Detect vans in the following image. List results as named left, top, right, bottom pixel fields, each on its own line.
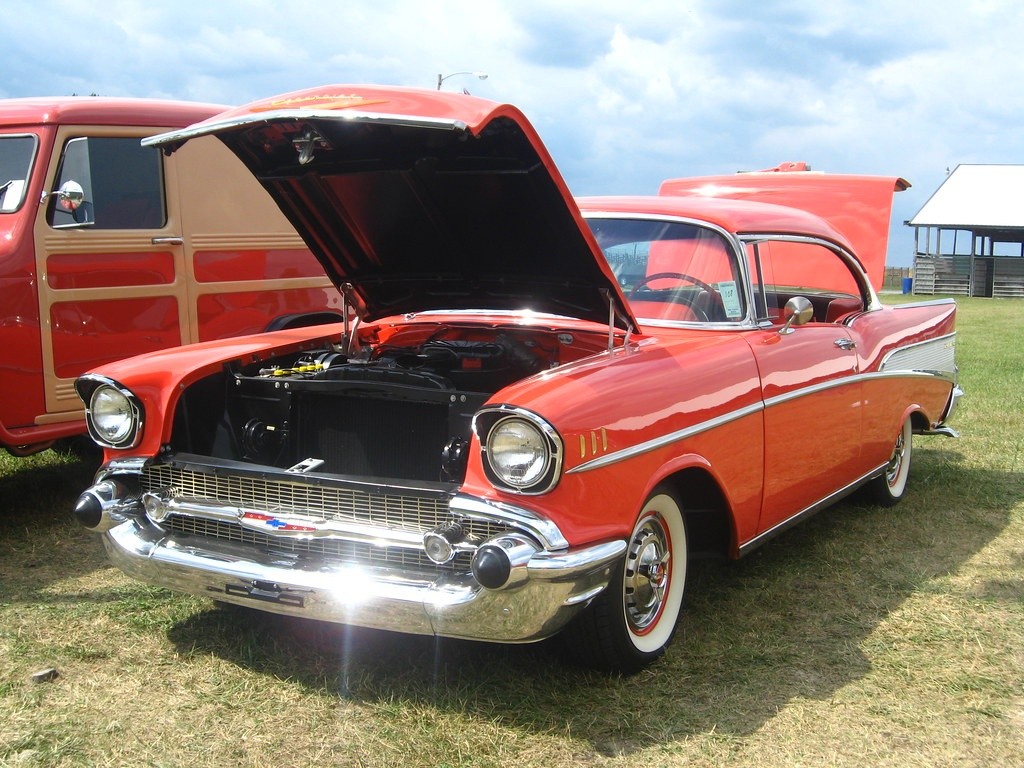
left=0, top=98, right=355, bottom=460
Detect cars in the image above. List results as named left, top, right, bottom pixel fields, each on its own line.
left=74, top=84, right=963, bottom=676
left=616, top=262, right=647, bottom=287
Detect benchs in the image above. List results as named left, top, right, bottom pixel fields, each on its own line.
left=624, top=290, right=862, bottom=322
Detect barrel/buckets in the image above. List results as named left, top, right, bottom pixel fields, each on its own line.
left=903, top=278, right=912, bottom=295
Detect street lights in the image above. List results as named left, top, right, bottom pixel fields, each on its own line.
left=436, top=71, right=489, bottom=89
